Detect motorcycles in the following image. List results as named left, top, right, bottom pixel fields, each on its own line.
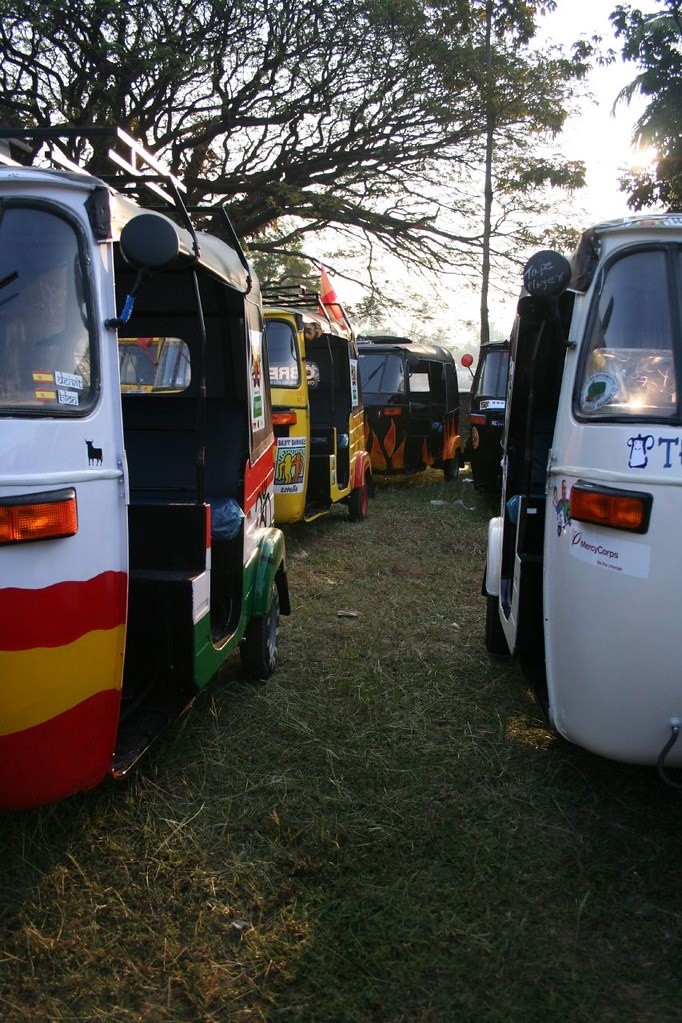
left=458, top=338, right=514, bottom=493
left=477, top=214, right=682, bottom=777
left=1, top=131, right=293, bottom=793
left=101, top=283, right=380, bottom=527
left=352, top=335, right=466, bottom=479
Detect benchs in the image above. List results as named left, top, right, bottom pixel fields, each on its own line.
left=515, top=496, right=547, bottom=654
left=128, top=503, right=210, bottom=691
left=309, top=427, right=337, bottom=504
left=121, top=394, right=247, bottom=539
left=409, top=397, right=445, bottom=463
left=508, top=391, right=560, bottom=529
left=308, top=388, right=348, bottom=450
